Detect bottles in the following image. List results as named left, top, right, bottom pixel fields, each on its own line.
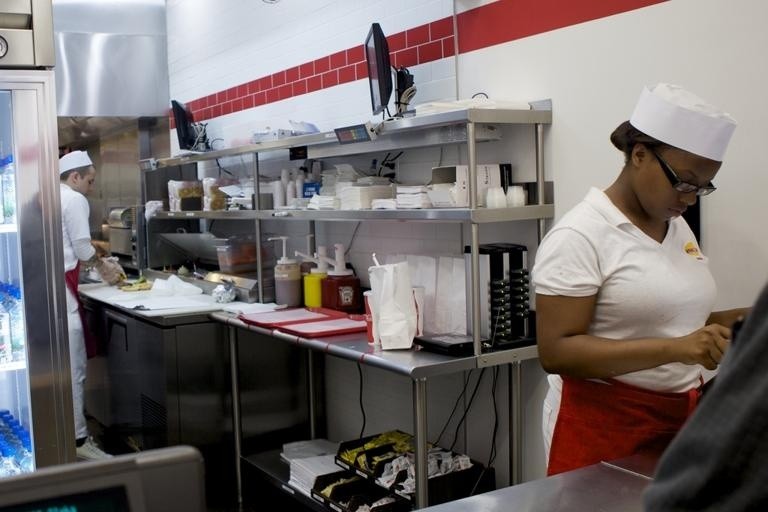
left=198, top=137, right=205, bottom=152
left=0, top=150, right=39, bottom=482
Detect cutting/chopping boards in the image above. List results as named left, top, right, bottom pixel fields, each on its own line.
left=76, top=274, right=226, bottom=317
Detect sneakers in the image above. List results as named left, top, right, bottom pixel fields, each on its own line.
left=74, top=436, right=112, bottom=463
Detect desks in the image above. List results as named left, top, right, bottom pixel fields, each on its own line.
left=409, top=462, right=654, bottom=512
left=601, top=445, right=664, bottom=480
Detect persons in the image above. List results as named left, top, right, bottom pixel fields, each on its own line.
left=529, top=81, right=737, bottom=477
left=60, top=149, right=127, bottom=463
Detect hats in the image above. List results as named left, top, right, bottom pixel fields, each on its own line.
left=630, top=84, right=740, bottom=160
left=54, top=149, right=96, bottom=174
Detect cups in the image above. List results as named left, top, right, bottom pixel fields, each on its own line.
left=270, top=163, right=322, bottom=211
left=411, top=286, right=425, bottom=338
left=362, top=287, right=383, bottom=345
left=486, top=184, right=525, bottom=209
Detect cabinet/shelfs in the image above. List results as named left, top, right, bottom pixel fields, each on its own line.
left=136, top=100, right=554, bottom=511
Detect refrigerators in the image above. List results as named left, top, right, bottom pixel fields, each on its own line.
left=0, top=65, right=84, bottom=491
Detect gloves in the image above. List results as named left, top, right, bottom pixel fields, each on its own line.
left=94, top=256, right=127, bottom=285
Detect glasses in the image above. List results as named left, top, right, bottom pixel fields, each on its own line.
left=651, top=149, right=719, bottom=196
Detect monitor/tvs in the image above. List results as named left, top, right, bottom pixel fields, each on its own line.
left=172, top=100, right=210, bottom=151
left=365, top=23, right=416, bottom=118
